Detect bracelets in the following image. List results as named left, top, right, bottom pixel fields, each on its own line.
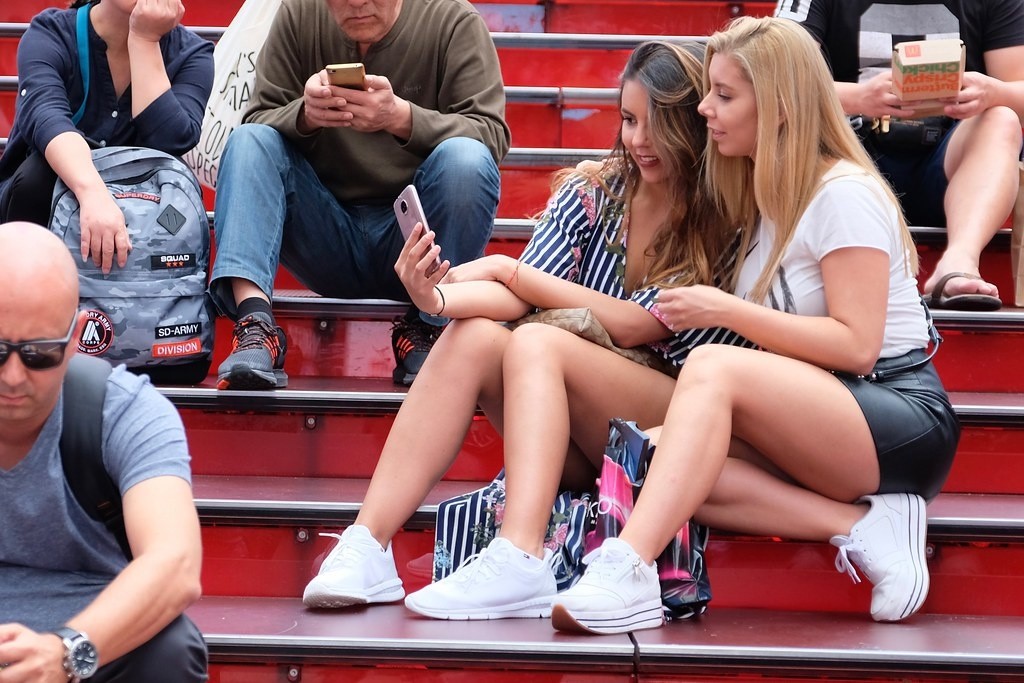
left=506, top=262, right=524, bottom=288
left=424, top=285, right=446, bottom=316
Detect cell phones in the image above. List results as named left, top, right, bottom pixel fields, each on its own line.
left=325, top=63, right=368, bottom=92
left=392, top=184, right=441, bottom=279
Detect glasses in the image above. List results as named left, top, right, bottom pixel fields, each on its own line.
left=0, top=309, right=78, bottom=370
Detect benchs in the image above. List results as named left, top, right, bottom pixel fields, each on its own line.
left=0, top=0, right=1024, bottom=683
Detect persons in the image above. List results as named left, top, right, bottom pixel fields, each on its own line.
left=551, top=15, right=963, bottom=636
left=209, top=0, right=512, bottom=391
left=0, top=0, right=215, bottom=275
left=302, top=40, right=768, bottom=621
left=0, top=222, right=209, bottom=683
left=773, top=0, right=1024, bottom=310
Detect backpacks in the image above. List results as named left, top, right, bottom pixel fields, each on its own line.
left=49, top=145, right=214, bottom=383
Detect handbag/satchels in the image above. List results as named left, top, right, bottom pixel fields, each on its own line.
left=182, top=0, right=283, bottom=190
left=432, top=466, right=590, bottom=597
left=582, top=416, right=712, bottom=622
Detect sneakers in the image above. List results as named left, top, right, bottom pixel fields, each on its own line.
left=303, top=524, right=405, bottom=607
left=216, top=315, right=289, bottom=386
left=404, top=538, right=557, bottom=619
left=831, top=492, right=930, bottom=621
left=389, top=314, right=443, bottom=383
left=551, top=538, right=664, bottom=633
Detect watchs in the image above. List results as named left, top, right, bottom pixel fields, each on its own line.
left=53, top=627, right=98, bottom=683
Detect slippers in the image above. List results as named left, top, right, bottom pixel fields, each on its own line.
left=922, top=272, right=1002, bottom=310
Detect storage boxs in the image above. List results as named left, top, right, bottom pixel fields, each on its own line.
left=892, top=38, right=967, bottom=118
